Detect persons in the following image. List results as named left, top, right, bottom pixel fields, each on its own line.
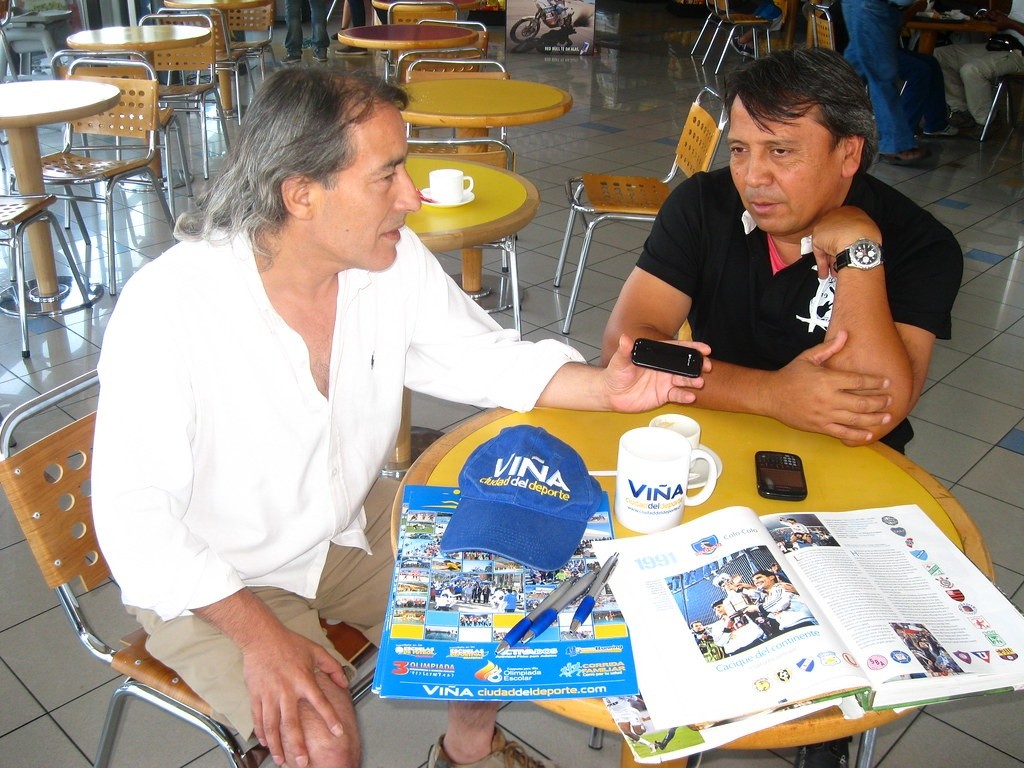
left=776, top=517, right=841, bottom=555
left=898, top=0, right=959, bottom=135
left=933, top=0, right=1024, bottom=141
left=331, top=0, right=351, bottom=40
left=601, top=45, right=964, bottom=768
left=730, top=0, right=783, bottom=58
left=690, top=563, right=817, bottom=659
left=603, top=693, right=707, bottom=754
left=335, top=0, right=388, bottom=54
left=393, top=513, right=517, bottom=626
left=280, top=0, right=330, bottom=64
left=90, top=56, right=712, bottom=767
left=524, top=514, right=592, bottom=638
left=840, top=0, right=932, bottom=165
left=894, top=624, right=962, bottom=676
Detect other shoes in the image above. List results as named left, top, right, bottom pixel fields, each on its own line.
left=331, top=33, right=339, bottom=39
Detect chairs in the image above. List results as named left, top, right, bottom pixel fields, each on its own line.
left=689, top=0, right=1024, bottom=142
left=0, top=0, right=522, bottom=359
left=553, top=87, right=730, bottom=335
left=0, top=370, right=380, bottom=768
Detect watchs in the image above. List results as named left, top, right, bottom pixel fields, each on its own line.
left=833, top=236, right=884, bottom=274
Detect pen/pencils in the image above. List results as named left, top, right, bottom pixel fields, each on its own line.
left=521, top=569, right=598, bottom=644
left=569, top=552, right=619, bottom=634
left=495, top=577, right=576, bottom=655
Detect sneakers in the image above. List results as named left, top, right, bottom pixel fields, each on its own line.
left=967, top=116, right=1002, bottom=141
left=948, top=110, right=975, bottom=128
left=923, top=124, right=959, bottom=138
left=729, top=36, right=744, bottom=54
left=741, top=45, right=755, bottom=58
left=430, top=725, right=555, bottom=768
left=313, top=50, right=327, bottom=61
left=796, top=734, right=853, bottom=768
left=879, top=147, right=931, bottom=165
left=280, top=54, right=301, bottom=64
left=335, top=45, right=368, bottom=55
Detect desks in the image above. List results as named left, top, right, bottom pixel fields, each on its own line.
left=905, top=15, right=998, bottom=55
left=1, top=0, right=571, bottom=314
left=389, top=405, right=993, bottom=768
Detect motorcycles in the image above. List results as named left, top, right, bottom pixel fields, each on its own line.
left=509, top=0, right=578, bottom=44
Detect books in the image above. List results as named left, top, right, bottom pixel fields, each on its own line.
left=591, top=502, right=1023, bottom=730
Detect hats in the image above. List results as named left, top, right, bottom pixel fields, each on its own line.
left=439, top=424, right=603, bottom=572
left=712, top=573, right=732, bottom=588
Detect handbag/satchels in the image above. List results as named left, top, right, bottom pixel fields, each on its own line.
left=986, top=33, right=1024, bottom=56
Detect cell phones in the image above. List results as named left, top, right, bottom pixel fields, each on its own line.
left=755, top=451, right=808, bottom=501
left=631, top=338, right=703, bottom=378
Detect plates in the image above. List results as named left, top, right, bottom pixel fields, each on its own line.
left=685, top=445, right=722, bottom=490
left=418, top=187, right=476, bottom=208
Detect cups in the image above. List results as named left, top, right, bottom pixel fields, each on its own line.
left=429, top=168, right=474, bottom=205
left=648, top=414, right=701, bottom=468
left=613, top=426, right=718, bottom=535
